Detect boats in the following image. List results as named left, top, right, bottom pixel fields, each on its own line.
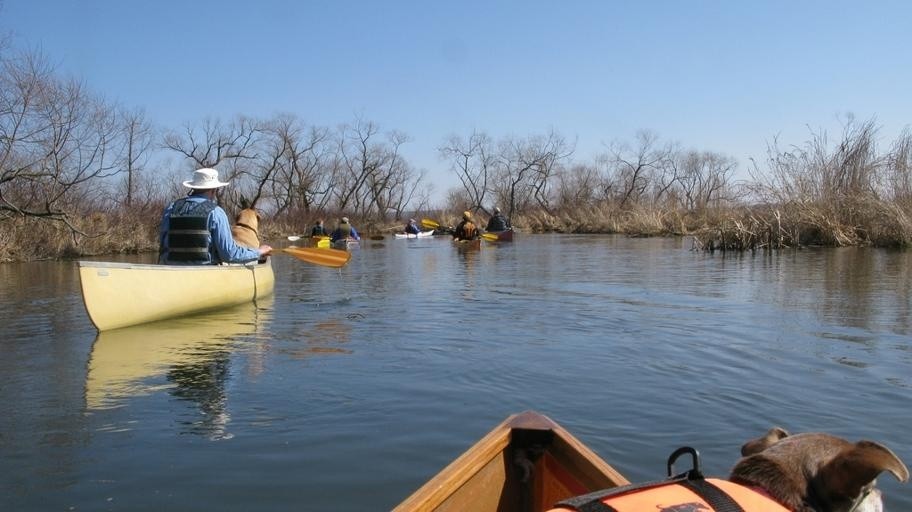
left=384, top=407, right=638, bottom=512
left=75, top=251, right=276, bottom=332
left=310, top=215, right=515, bottom=255
left=81, top=291, right=276, bottom=414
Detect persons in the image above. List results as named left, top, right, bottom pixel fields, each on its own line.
left=405, top=219, right=420, bottom=234
left=331, top=216, right=360, bottom=242
left=159, top=168, right=273, bottom=266
left=455, top=211, right=479, bottom=241
left=311, top=220, right=328, bottom=237
left=485, top=207, right=508, bottom=232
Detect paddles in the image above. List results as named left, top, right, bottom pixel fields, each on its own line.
left=318, top=237, right=359, bottom=248
left=421, top=218, right=499, bottom=242
left=268, top=248, right=353, bottom=267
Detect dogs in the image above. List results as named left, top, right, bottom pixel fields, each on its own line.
left=727, top=426, right=910, bottom=512
left=219, top=208, right=262, bottom=266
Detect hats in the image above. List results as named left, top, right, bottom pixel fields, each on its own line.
left=341, top=217, right=349, bottom=223
left=183, top=168, right=229, bottom=189
left=409, top=219, right=416, bottom=224
left=464, top=210, right=473, bottom=219
left=492, top=207, right=501, bottom=212
